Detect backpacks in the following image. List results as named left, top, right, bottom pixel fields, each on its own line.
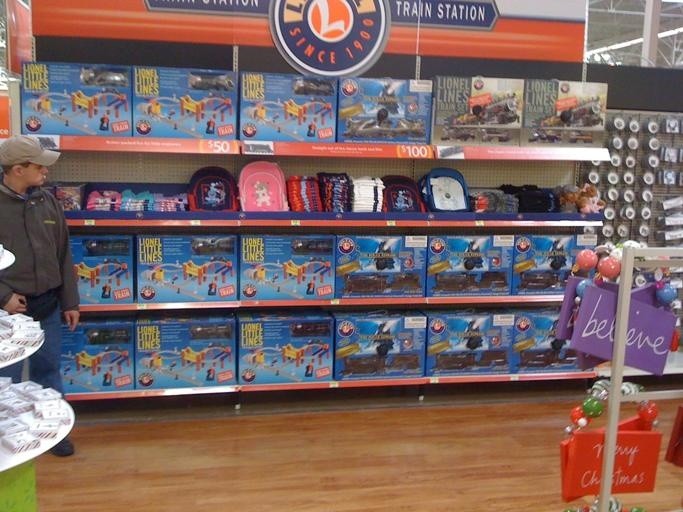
left=417, top=165, right=470, bottom=213
left=378, top=173, right=424, bottom=216
left=237, top=159, right=288, bottom=213
left=185, top=163, right=239, bottom=215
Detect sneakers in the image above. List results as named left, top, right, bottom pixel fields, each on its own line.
left=52, top=438, right=75, bottom=458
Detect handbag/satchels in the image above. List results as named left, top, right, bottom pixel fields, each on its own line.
left=21, top=289, right=64, bottom=322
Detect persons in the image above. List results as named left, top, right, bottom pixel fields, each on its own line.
left=0, top=131, right=81, bottom=457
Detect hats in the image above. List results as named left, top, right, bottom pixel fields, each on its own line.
left=0, top=135, right=59, bottom=167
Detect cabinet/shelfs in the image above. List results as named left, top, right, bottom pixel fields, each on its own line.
left=18, top=135, right=610, bottom=401
